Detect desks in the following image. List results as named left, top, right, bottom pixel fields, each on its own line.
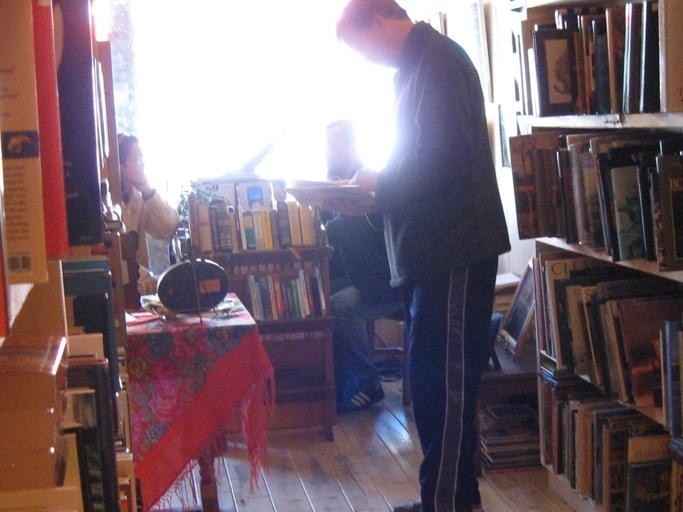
left=472, top=339, right=541, bottom=478
left=124, top=292, right=277, bottom=512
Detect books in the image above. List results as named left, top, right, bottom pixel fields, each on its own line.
left=513, top=2, right=681, bottom=117
left=509, top=131, right=682, bottom=273
left=288, top=180, right=373, bottom=205
left=479, top=245, right=681, bottom=510
left=187, top=191, right=326, bottom=321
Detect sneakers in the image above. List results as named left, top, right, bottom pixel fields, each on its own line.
left=338, top=384, right=384, bottom=413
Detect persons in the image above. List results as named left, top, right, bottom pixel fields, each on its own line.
left=337, top=0, right=512, bottom=512
left=116, top=132, right=179, bottom=295
left=318, top=120, right=405, bottom=414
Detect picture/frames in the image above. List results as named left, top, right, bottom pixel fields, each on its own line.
left=496, top=260, right=535, bottom=357
left=532, top=30, right=572, bottom=117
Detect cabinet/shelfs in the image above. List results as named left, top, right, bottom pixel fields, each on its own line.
left=0, top=0, right=140, bottom=512
left=509, top=0, right=683, bottom=512
left=171, top=238, right=337, bottom=442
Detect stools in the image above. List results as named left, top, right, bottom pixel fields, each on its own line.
left=367, top=310, right=410, bottom=405
